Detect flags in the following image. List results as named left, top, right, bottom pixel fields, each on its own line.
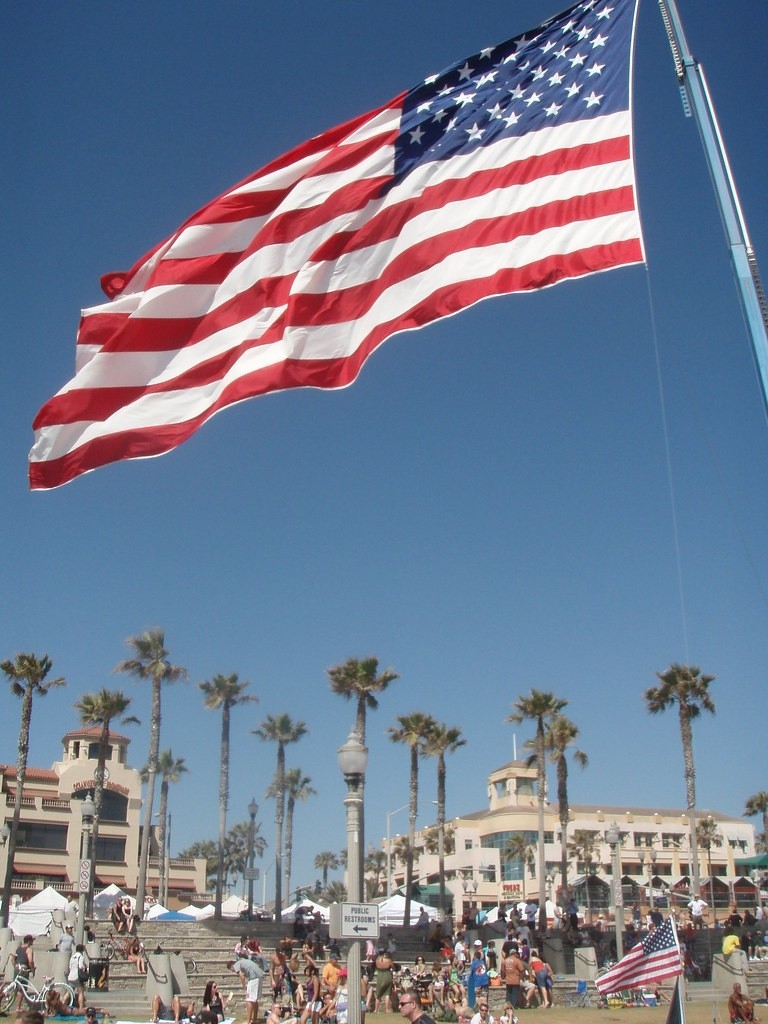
left=26, top=1, right=648, bottom=492
left=594, top=909, right=685, bottom=995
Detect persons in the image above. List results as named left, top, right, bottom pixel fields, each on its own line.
left=413, top=900, right=555, bottom=1009
left=203, top=981, right=224, bottom=1024
left=751, top=865, right=765, bottom=886
left=293, top=905, right=342, bottom=968
left=566, top=899, right=579, bottom=927
left=111, top=896, right=126, bottom=932
left=68, top=944, right=86, bottom=1010
left=41, top=990, right=116, bottom=1018
left=11, top=935, right=35, bottom=1014
left=227, top=958, right=266, bottom=1024
left=152, top=994, right=196, bottom=1024
left=499, top=1002, right=519, bottom=1024
left=470, top=1003, right=497, bottom=1024
left=266, top=936, right=342, bottom=1024
left=234, top=934, right=269, bottom=973
left=553, top=902, right=562, bottom=929
left=22, top=1011, right=44, bottom=1024
left=122, top=898, right=134, bottom=933
left=56, top=925, right=76, bottom=979
left=728, top=983, right=759, bottom=1024
left=596, top=893, right=768, bottom=1010
left=398, top=991, right=436, bottom=1024
left=64, top=894, right=79, bottom=920
left=326, top=935, right=465, bottom=1024
left=85, top=1007, right=98, bottom=1024
left=84, top=925, right=95, bottom=943
left=126, top=936, right=146, bottom=974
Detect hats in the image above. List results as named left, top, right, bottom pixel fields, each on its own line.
left=23, top=935, right=36, bottom=943
left=65, top=925, right=73, bottom=929
left=509, top=949, right=517, bottom=955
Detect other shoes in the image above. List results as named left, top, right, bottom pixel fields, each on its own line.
left=748, top=956, right=768, bottom=961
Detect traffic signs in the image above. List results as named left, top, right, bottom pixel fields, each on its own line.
left=340, top=902, right=380, bottom=938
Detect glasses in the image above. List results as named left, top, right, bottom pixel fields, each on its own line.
left=399, top=1002, right=411, bottom=1007
left=275, top=1007, right=282, bottom=1010
left=480, top=1008, right=488, bottom=1012
left=213, top=985, right=217, bottom=989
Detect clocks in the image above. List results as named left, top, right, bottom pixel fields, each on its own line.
left=94, top=766, right=110, bottom=781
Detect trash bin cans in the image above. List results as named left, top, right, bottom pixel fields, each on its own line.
left=87, top=956, right=108, bottom=992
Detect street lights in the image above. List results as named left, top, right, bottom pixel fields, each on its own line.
left=386, top=801, right=439, bottom=897
left=335, top=725, right=370, bottom=1024
left=497, top=867, right=520, bottom=909
left=606, top=825, right=624, bottom=964
left=461, top=879, right=479, bottom=909
left=262, top=854, right=285, bottom=911
left=247, top=797, right=260, bottom=922
left=75, top=790, right=97, bottom=950
left=637, top=849, right=657, bottom=908
left=154, top=809, right=171, bottom=910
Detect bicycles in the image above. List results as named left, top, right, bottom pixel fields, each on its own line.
left=99, top=926, right=146, bottom=960
left=155, top=941, right=197, bottom=975
left=0, top=962, right=75, bottom=1016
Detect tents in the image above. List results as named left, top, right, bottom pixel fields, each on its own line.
left=481, top=900, right=585, bottom=924
left=10, top=883, right=440, bottom=938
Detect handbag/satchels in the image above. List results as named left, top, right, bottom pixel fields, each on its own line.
left=389, top=990, right=400, bottom=1011
left=133, top=915, right=140, bottom=922
left=412, top=965, right=418, bottom=982
left=77, top=968, right=89, bottom=982
